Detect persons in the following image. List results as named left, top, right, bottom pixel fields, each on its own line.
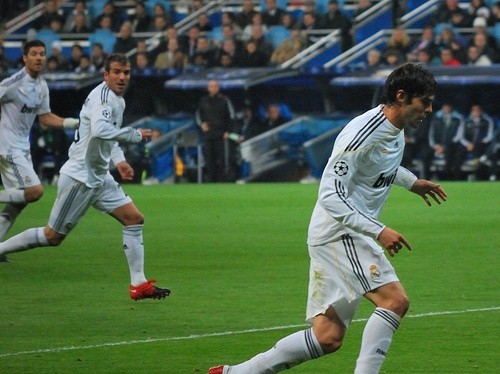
left=198, top=80, right=236, bottom=183
left=0, top=55, right=171, bottom=302
left=2, top=1, right=500, bottom=183
left=0, top=40, right=81, bottom=261
left=211, top=63, right=447, bottom=374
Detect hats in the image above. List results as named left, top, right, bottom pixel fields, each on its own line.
left=477, top=7, right=489, bottom=17
left=473, top=17, right=486, bottom=27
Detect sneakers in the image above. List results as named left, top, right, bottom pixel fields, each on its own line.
left=208, top=364, right=230, bottom=374
left=129, top=279, right=171, bottom=301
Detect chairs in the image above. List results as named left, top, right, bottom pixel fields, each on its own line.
left=0, top=0, right=500, bottom=180
